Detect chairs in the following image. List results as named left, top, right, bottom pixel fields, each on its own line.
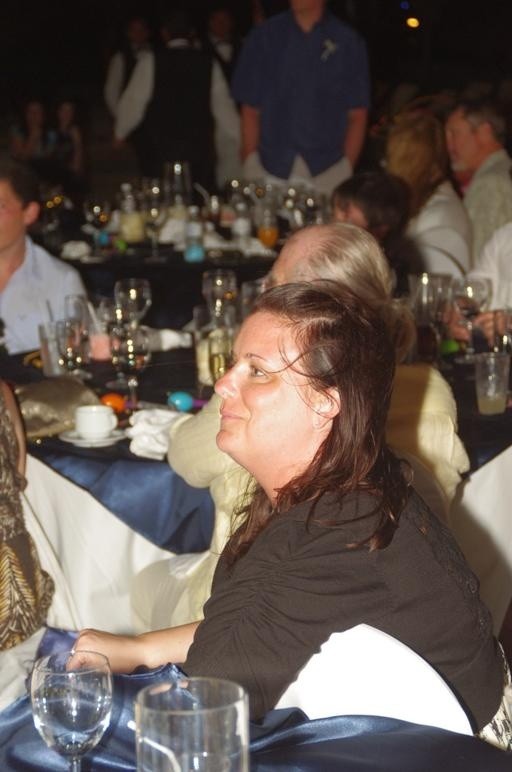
left=270, top=623, right=474, bottom=738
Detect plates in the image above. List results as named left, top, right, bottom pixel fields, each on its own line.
left=57, top=429, right=128, bottom=449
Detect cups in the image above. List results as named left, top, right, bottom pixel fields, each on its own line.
left=476, top=353, right=510, bottom=414
left=74, top=404, right=117, bottom=438
left=134, top=677, right=251, bottom=770
left=190, top=267, right=279, bottom=386
left=38, top=322, right=65, bottom=375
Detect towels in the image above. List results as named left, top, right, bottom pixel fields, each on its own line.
left=122, top=401, right=196, bottom=461
left=144, top=326, right=194, bottom=355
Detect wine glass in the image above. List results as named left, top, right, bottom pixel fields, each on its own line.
left=30, top=652, right=116, bottom=771
left=84, top=160, right=327, bottom=264
left=413, top=271, right=489, bottom=366
left=59, top=291, right=96, bottom=381
left=110, top=279, right=152, bottom=406
